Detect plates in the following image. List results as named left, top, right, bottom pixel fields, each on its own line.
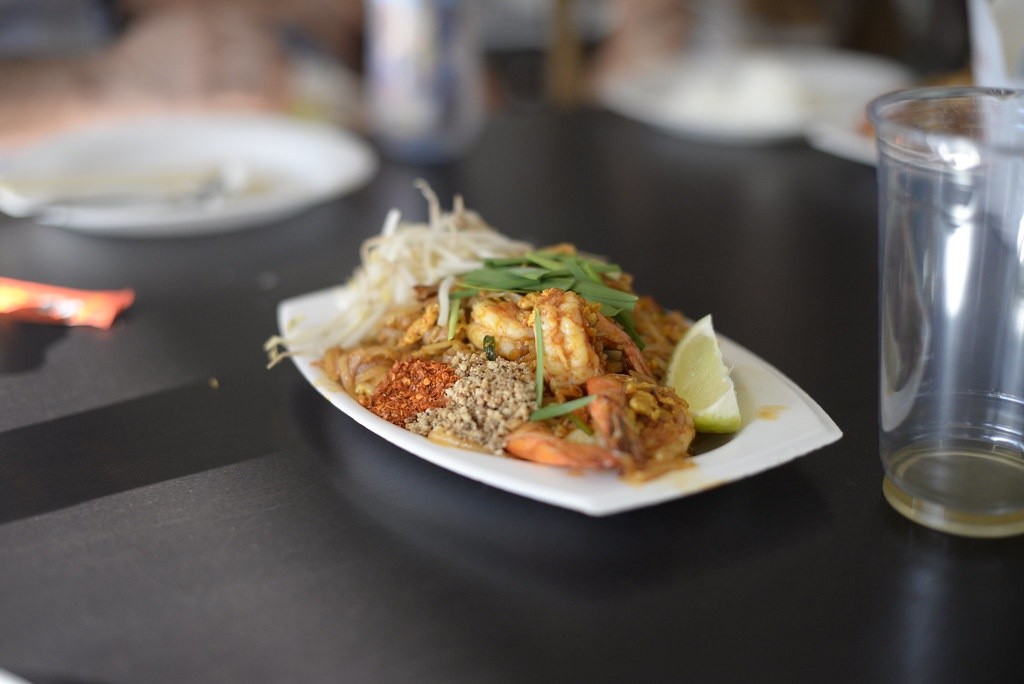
left=0, top=110, right=383, bottom=240
left=273, top=277, right=843, bottom=518
left=600, top=42, right=913, bottom=146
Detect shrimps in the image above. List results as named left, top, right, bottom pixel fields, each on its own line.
left=466, top=287, right=696, bottom=470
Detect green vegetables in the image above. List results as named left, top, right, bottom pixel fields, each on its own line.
left=435, top=244, right=647, bottom=418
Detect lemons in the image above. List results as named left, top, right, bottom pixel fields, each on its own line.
left=659, top=312, right=741, bottom=432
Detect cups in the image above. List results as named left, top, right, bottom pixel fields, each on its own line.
left=866, top=86, right=1024, bottom=537
